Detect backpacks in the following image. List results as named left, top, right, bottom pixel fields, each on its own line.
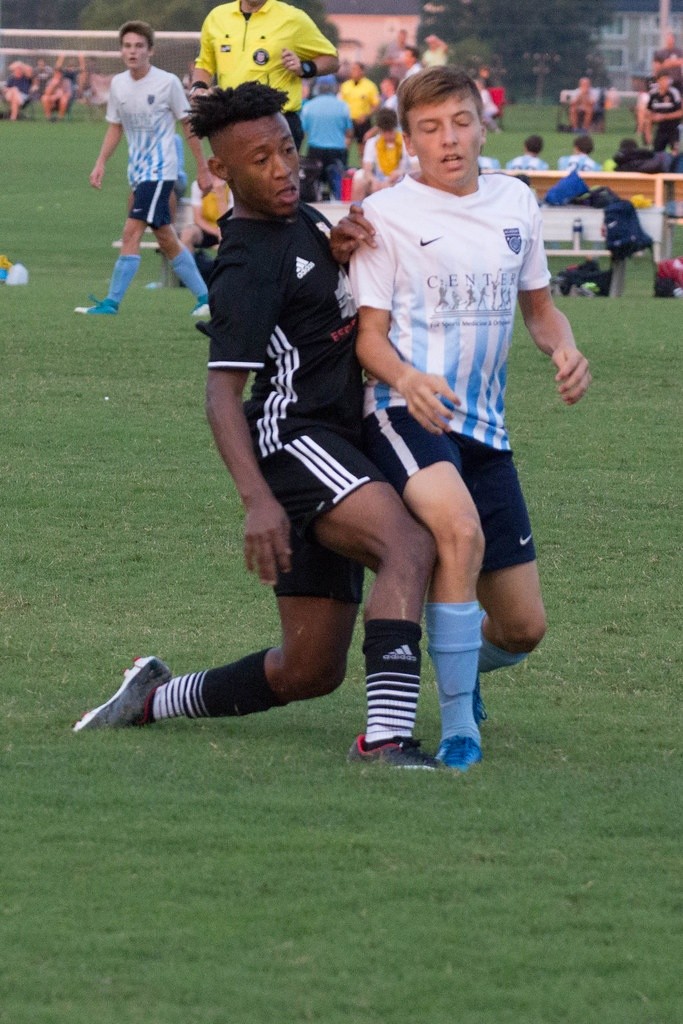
left=326, top=159, right=350, bottom=199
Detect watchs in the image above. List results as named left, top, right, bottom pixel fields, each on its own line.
left=300, top=61, right=311, bottom=78
left=189, top=86, right=198, bottom=97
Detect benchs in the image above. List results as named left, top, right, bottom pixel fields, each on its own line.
left=540, top=205, right=631, bottom=298
left=112, top=199, right=348, bottom=292
left=483, top=168, right=683, bottom=264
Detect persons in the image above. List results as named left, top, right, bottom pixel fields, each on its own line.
left=0, top=0, right=683, bottom=316
left=349, top=65, right=593, bottom=770
left=73, top=83, right=446, bottom=768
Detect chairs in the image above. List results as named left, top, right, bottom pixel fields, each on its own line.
left=491, top=86, right=507, bottom=133
left=0, top=68, right=79, bottom=120
left=556, top=87, right=604, bottom=134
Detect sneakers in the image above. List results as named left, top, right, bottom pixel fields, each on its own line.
left=73, top=295, right=120, bottom=316
left=73, top=654, right=171, bottom=735
left=467, top=675, right=490, bottom=728
left=436, top=732, right=483, bottom=773
left=349, top=732, right=437, bottom=773
left=188, top=301, right=211, bottom=317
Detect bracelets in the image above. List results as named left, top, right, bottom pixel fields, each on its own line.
left=307, top=60, right=316, bottom=77
left=192, top=81, right=209, bottom=88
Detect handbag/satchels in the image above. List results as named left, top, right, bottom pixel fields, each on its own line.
left=541, top=162, right=683, bottom=298
left=295, top=154, right=321, bottom=202
